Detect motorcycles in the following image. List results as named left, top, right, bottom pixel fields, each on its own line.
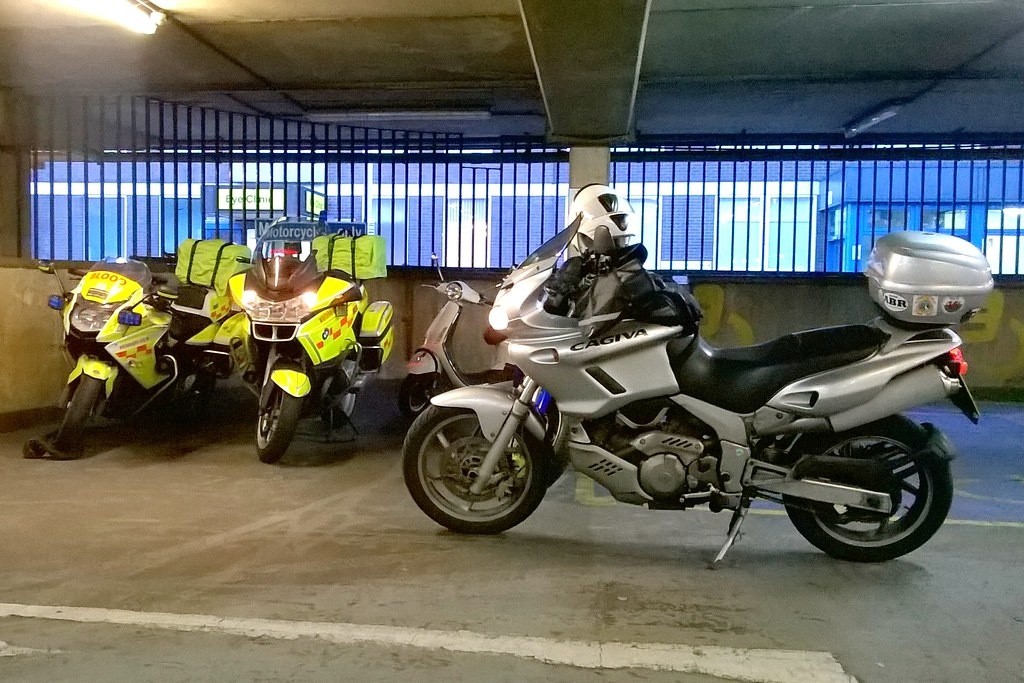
left=397, top=250, right=523, bottom=434
left=33, top=258, right=248, bottom=465
left=397, top=182, right=999, bottom=569
left=201, top=209, right=396, bottom=468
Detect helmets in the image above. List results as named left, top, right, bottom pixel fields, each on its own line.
left=567, top=183, right=636, bottom=254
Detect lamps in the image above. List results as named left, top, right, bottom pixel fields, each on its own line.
left=305, top=105, right=493, bottom=121
left=843, top=100, right=903, bottom=139
